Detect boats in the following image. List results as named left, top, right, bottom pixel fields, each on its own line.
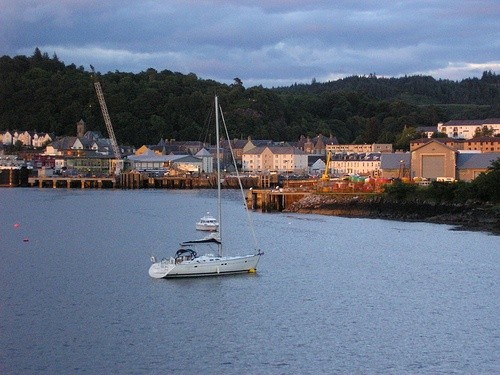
left=195, top=211, right=220, bottom=232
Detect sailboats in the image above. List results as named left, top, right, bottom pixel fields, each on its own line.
left=148, top=95, right=265, bottom=280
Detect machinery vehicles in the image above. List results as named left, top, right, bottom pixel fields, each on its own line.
left=88, top=63, right=133, bottom=176
left=319, top=149, right=333, bottom=182
